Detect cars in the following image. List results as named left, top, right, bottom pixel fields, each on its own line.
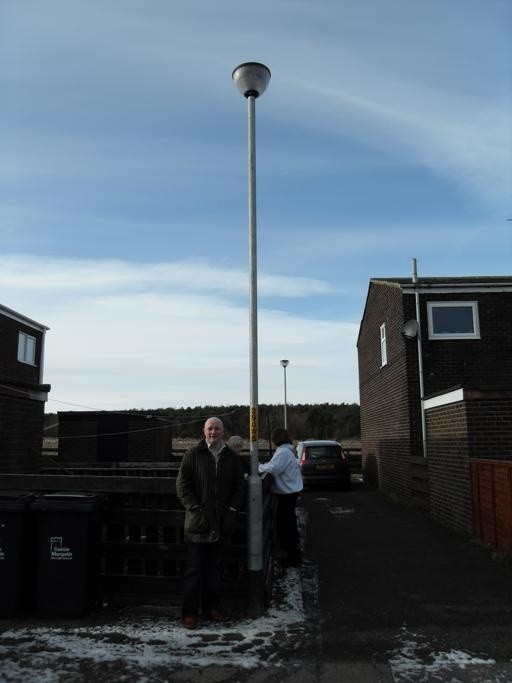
left=295, top=439, right=352, bottom=491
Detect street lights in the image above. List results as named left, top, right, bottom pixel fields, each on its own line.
left=232, top=61, right=271, bottom=618
left=280, top=359, right=288, bottom=430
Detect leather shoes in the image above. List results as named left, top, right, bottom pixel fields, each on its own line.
left=183, top=613, right=197, bottom=629
left=207, top=609, right=229, bottom=622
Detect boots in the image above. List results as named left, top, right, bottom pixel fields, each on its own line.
left=280, top=548, right=299, bottom=568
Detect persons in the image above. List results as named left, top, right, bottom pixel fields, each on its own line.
left=176, top=417, right=249, bottom=630
left=256, top=428, right=304, bottom=572
left=229, top=436, right=252, bottom=478
